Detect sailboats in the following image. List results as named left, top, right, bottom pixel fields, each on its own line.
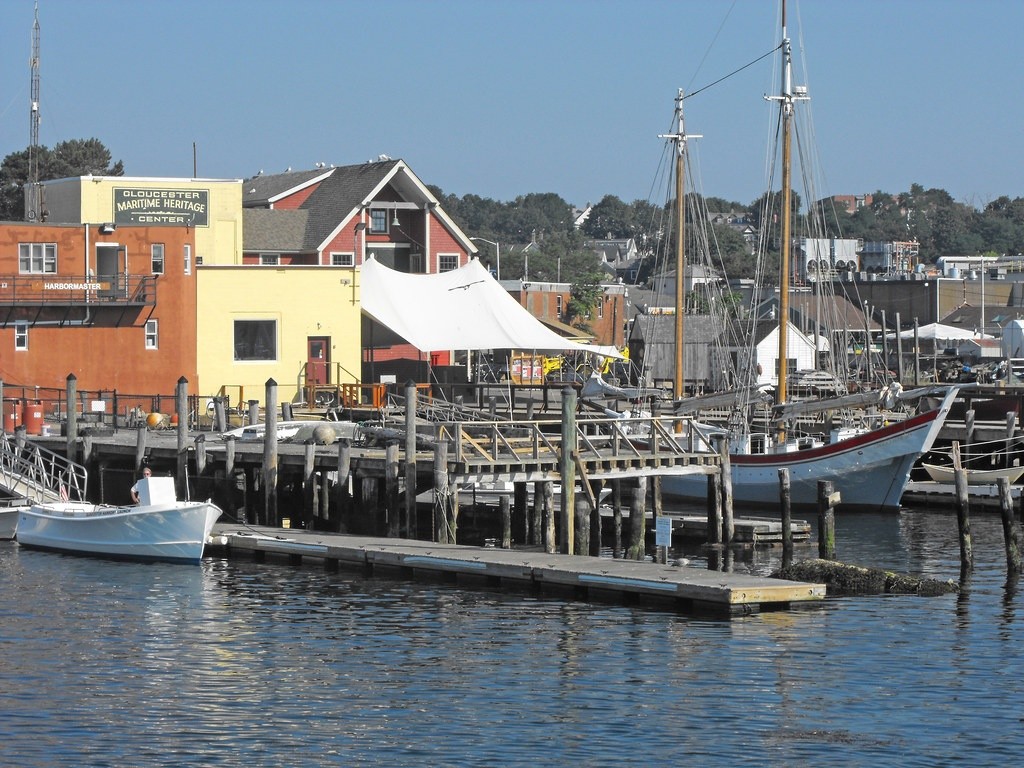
left=625, top=1, right=958, bottom=508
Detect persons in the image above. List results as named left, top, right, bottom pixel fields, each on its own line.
left=130, top=467, right=152, bottom=504
left=938, top=358, right=971, bottom=382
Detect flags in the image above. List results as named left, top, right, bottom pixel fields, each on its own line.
left=61, top=478, right=68, bottom=502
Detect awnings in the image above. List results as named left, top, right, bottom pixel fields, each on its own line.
left=360, top=252, right=646, bottom=410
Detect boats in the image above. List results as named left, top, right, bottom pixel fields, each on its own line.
left=921, top=462, right=1024, bottom=486
left=15, top=477, right=225, bottom=566
left=0, top=495, right=35, bottom=540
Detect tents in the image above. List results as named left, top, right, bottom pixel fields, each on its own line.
left=877, top=322, right=995, bottom=386
